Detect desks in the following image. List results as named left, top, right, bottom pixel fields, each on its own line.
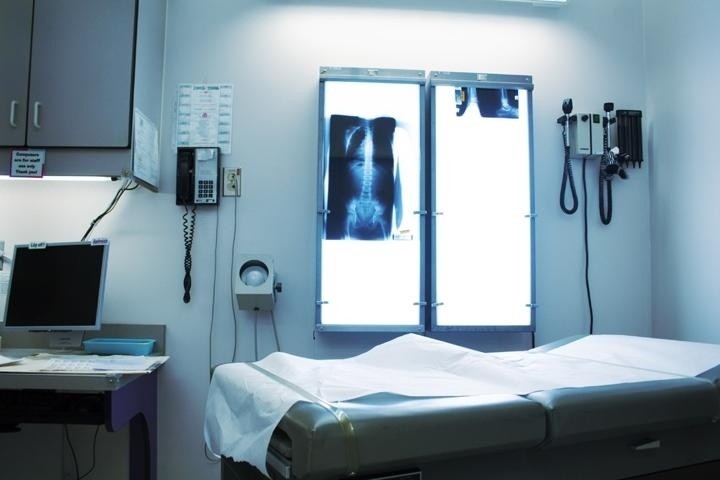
left=0, top=354, right=170, bottom=480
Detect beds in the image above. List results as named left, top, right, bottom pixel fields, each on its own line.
left=210, top=334, right=720, bottom=480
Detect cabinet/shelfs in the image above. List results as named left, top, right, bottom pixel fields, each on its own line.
left=0, top=1, right=167, bottom=192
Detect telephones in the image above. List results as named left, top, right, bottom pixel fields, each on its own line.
left=176, top=146, right=221, bottom=207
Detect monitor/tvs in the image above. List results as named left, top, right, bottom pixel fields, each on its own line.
left=1, top=237, right=110, bottom=349
